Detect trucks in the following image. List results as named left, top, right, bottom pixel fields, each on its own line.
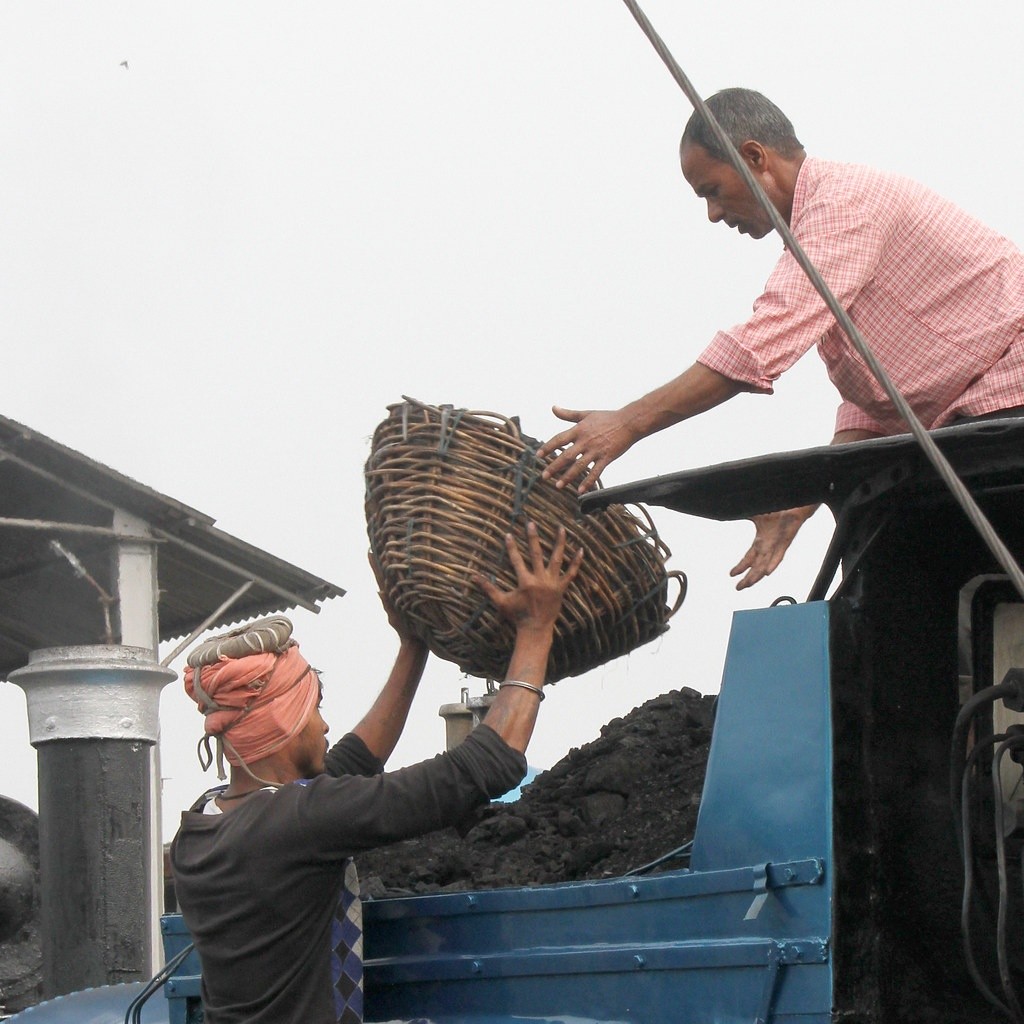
left=124, top=415, right=1020, bottom=1024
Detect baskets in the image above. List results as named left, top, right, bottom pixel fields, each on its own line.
left=363, top=396, right=687, bottom=688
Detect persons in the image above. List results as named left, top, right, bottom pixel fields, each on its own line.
left=536, top=86, right=1024, bottom=593
left=169, top=516, right=587, bottom=1023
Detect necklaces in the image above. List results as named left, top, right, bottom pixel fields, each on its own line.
left=214, top=790, right=254, bottom=800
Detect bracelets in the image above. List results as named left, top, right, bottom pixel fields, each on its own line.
left=499, top=681, right=546, bottom=702
